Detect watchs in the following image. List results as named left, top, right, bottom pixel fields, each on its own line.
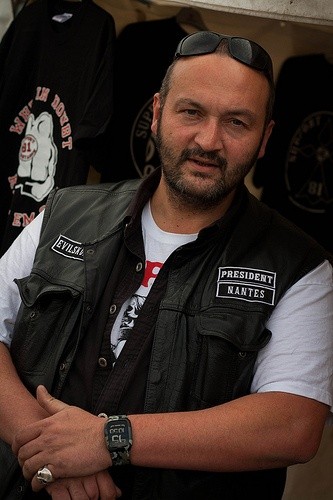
left=104, top=415, right=133, bottom=469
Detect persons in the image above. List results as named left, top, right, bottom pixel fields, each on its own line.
left=0, top=31, right=333, bottom=500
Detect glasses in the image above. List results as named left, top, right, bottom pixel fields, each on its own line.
left=174, top=30, right=274, bottom=88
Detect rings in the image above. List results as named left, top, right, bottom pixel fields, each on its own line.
left=37, top=465, right=54, bottom=486
